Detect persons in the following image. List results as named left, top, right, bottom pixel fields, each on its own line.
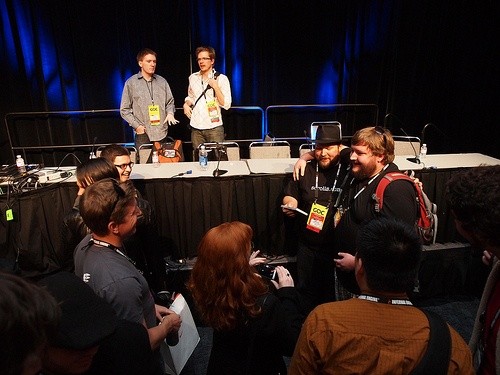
left=71, top=178, right=182, bottom=375
left=182, top=46, right=232, bottom=157
left=292, top=127, right=422, bottom=305
left=287, top=219, right=476, bottom=375
left=184, top=222, right=302, bottom=374
left=280, top=120, right=364, bottom=308
left=119, top=47, right=180, bottom=148
left=465, top=243, right=500, bottom=375
left=64, top=142, right=153, bottom=267
left=0, top=270, right=63, bottom=375
left=31, top=272, right=155, bottom=375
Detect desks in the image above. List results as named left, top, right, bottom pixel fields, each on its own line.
left=0, top=151, right=500, bottom=277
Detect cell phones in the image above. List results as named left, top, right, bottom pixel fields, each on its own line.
left=254, top=264, right=277, bottom=280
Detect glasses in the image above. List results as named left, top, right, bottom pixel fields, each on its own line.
left=197, top=58, right=210, bottom=61
left=108, top=179, right=124, bottom=220
left=114, top=161, right=134, bottom=170
left=374, top=127, right=387, bottom=149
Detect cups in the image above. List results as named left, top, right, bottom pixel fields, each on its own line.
left=162, top=316, right=180, bottom=347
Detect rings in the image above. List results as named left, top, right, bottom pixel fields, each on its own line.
left=286, top=274, right=289, bottom=276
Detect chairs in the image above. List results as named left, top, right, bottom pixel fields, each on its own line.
left=250, top=141, right=292, bottom=158
left=193, top=141, right=241, bottom=163
left=140, top=141, right=173, bottom=163
left=96, top=143, right=139, bottom=164
left=299, top=143, right=316, bottom=159
left=391, top=135, right=423, bottom=156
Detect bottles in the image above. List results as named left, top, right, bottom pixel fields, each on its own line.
left=152, top=152, right=158, bottom=168
left=16, top=154, right=26, bottom=173
left=90, top=152, right=96, bottom=159
left=420, top=144, right=427, bottom=159
left=199, top=146, right=207, bottom=171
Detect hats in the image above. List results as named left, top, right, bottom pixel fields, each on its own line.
left=309, top=124, right=348, bottom=145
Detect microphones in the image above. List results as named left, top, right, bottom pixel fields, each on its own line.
left=400, top=127, right=421, bottom=164
left=90, top=136, right=98, bottom=159
left=213, top=133, right=227, bottom=177
left=303, top=130, right=312, bottom=151
